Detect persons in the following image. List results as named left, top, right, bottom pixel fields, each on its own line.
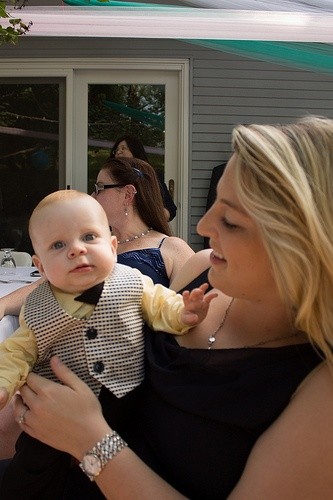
left=0, top=156, right=196, bottom=318
left=0, top=189, right=218, bottom=441
left=110, top=133, right=177, bottom=221
left=0, top=114, right=333, bottom=500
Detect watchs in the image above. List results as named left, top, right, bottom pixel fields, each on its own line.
left=79, top=431, right=128, bottom=481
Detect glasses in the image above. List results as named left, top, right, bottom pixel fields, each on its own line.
left=112, top=146, right=129, bottom=154
left=95, top=182, right=126, bottom=195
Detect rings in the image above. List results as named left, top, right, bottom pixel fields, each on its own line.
left=18, top=409, right=29, bottom=424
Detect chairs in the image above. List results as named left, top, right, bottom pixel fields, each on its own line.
left=0, top=251, right=32, bottom=267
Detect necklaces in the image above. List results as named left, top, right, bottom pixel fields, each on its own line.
left=118, top=228, right=152, bottom=244
left=208, top=296, right=303, bottom=350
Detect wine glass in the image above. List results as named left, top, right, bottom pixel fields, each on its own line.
left=0, top=248, right=17, bottom=275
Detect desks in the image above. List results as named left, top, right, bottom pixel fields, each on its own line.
left=0, top=266, right=45, bottom=462
